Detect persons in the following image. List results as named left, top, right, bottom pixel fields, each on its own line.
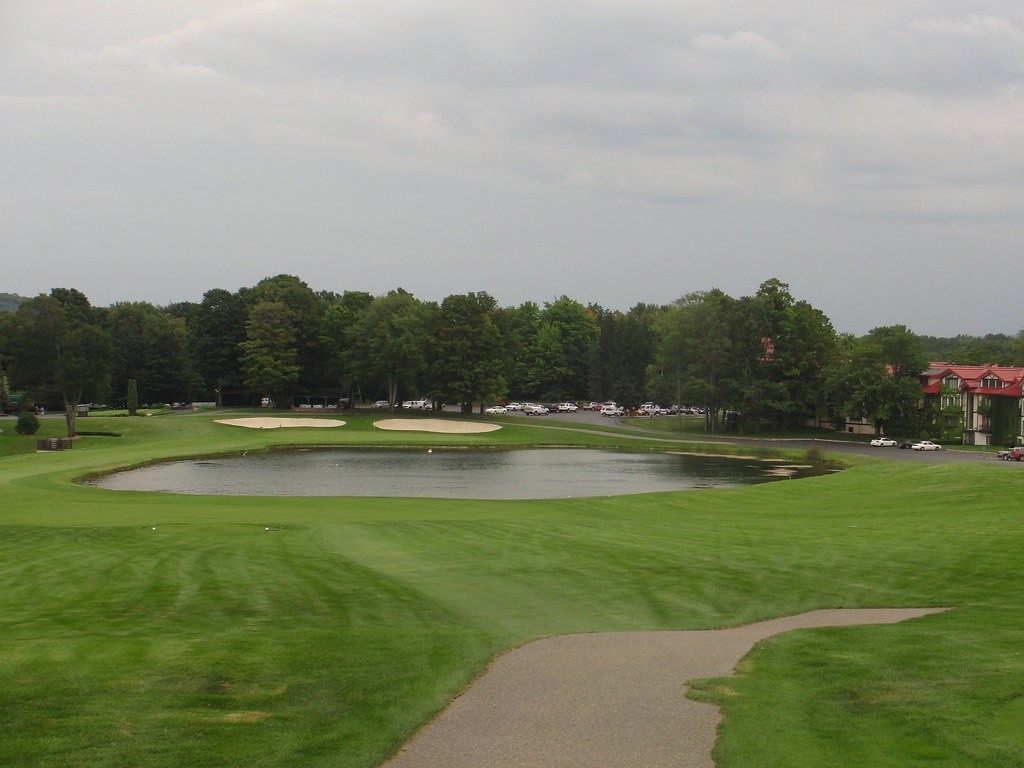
left=34, top=405, right=45, bottom=417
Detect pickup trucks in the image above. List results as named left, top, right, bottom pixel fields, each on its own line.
left=506, top=403, right=549, bottom=416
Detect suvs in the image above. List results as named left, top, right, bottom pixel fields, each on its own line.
left=558, top=403, right=578, bottom=413
left=583, top=401, right=617, bottom=411
left=626, top=402, right=740, bottom=416
left=600, top=407, right=625, bottom=417
left=371, top=401, right=390, bottom=408
left=998, top=447, right=1024, bottom=462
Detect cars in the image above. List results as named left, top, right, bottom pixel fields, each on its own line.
left=869, top=437, right=897, bottom=447
left=394, top=400, right=446, bottom=411
left=544, top=406, right=557, bottom=413
left=911, top=441, right=942, bottom=451
left=485, top=406, right=507, bottom=415
left=898, top=440, right=920, bottom=449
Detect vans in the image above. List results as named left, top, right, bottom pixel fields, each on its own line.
left=262, top=398, right=272, bottom=404
